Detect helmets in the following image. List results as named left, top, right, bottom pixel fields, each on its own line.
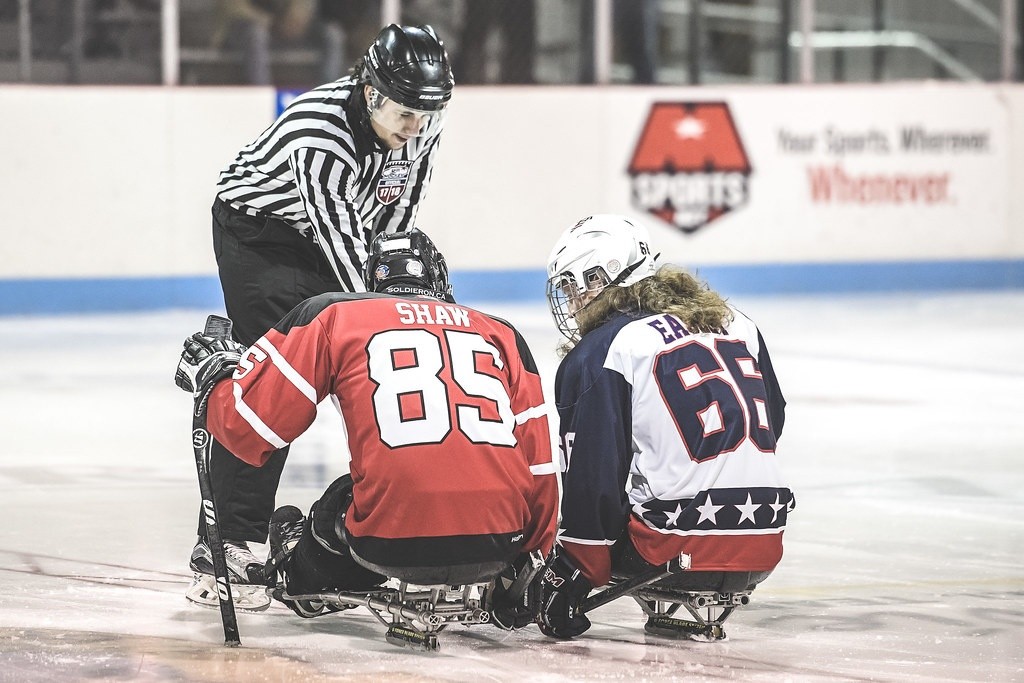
left=362, top=227, right=456, bottom=304
left=545, top=212, right=661, bottom=347
left=364, top=22, right=455, bottom=114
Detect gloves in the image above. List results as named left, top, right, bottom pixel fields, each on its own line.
left=538, top=552, right=592, bottom=641
left=173, top=332, right=249, bottom=398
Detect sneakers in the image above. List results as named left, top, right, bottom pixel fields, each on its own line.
left=185, top=535, right=271, bottom=614
left=269, top=504, right=347, bottom=618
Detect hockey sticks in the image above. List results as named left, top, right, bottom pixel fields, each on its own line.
left=582, top=553, right=690, bottom=613
left=192, top=313, right=241, bottom=645
left=510, top=550, right=544, bottom=605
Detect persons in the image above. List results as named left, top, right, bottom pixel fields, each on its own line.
left=472, top=212, right=799, bottom=643
left=184, top=22, right=458, bottom=613
left=174, top=227, right=560, bottom=652
